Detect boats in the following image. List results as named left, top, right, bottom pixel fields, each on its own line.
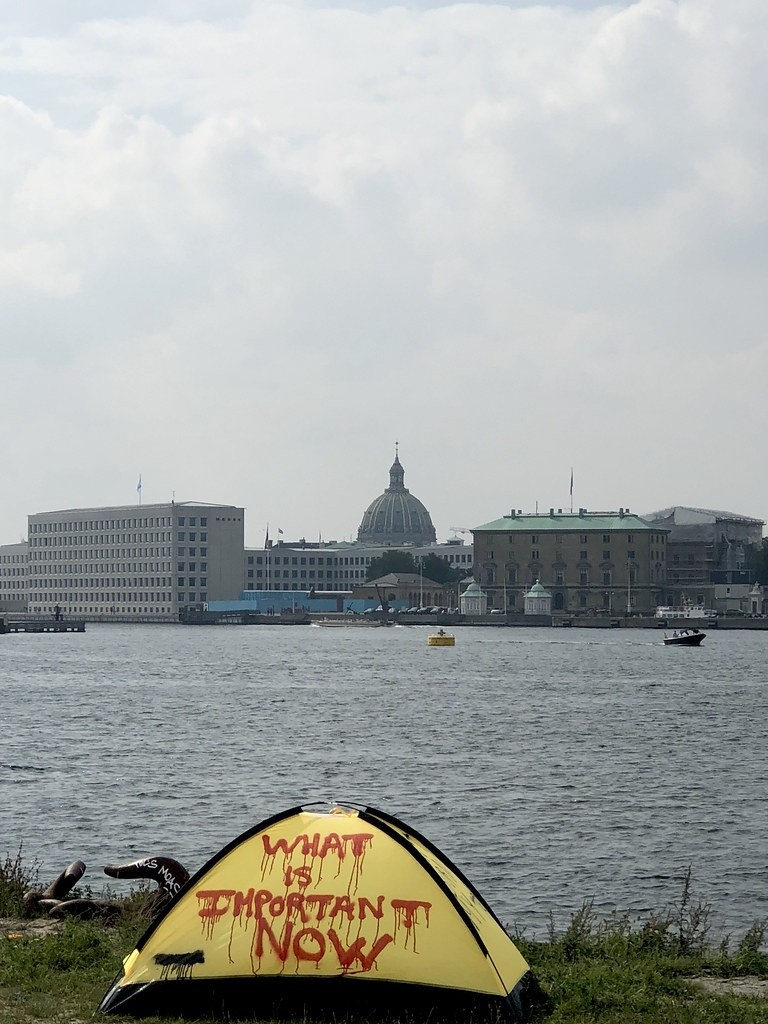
left=663, top=628, right=706, bottom=645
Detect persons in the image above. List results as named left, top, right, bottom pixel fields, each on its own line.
left=673, top=628, right=700, bottom=638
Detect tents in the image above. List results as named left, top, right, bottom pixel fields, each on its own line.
left=96, top=798, right=553, bottom=1024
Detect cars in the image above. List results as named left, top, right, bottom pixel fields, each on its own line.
left=398, top=607, right=408, bottom=615
left=364, top=607, right=376, bottom=615
left=429, top=607, right=444, bottom=615
left=417, top=606, right=436, bottom=615
left=375, top=605, right=395, bottom=612
left=441, top=608, right=454, bottom=616
left=407, top=606, right=421, bottom=614
left=491, top=607, right=505, bottom=614
